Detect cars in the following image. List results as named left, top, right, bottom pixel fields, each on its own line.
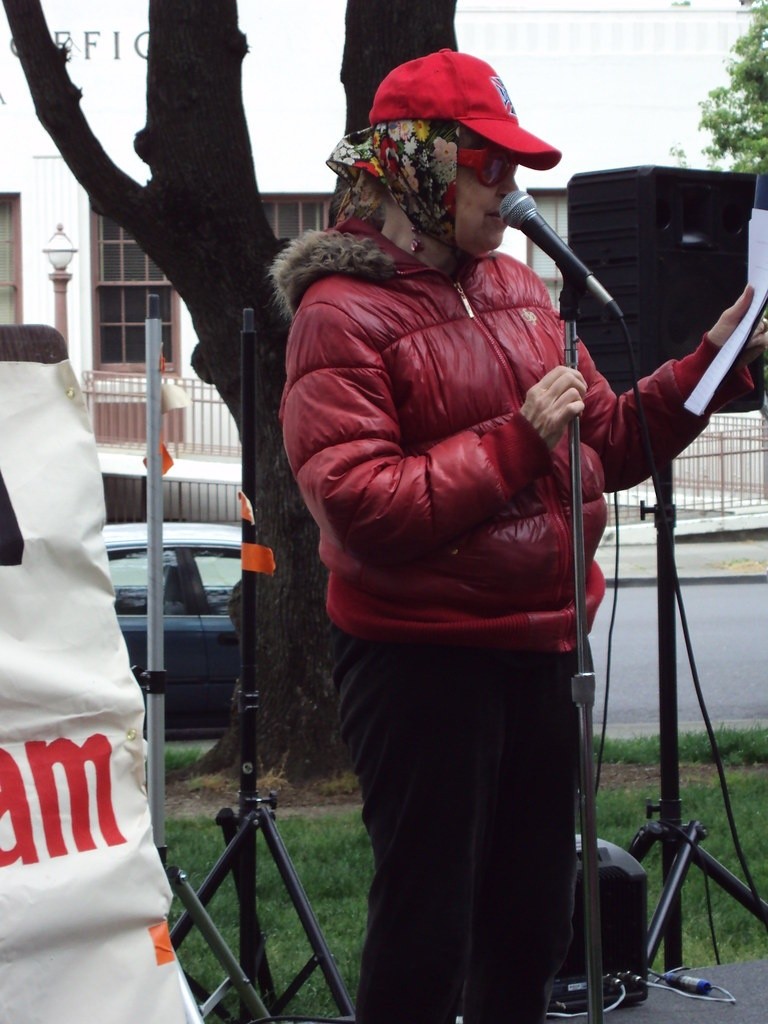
left=105, top=521, right=241, bottom=727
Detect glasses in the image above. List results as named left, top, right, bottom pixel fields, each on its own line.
left=455, top=143, right=518, bottom=187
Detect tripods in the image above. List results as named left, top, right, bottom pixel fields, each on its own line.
left=173, top=306, right=354, bottom=1020
left=625, top=464, right=768, bottom=973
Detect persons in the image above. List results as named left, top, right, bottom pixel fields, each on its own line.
left=268, top=50, right=768, bottom=1024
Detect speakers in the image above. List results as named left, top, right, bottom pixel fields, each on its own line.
left=548, top=835, right=648, bottom=1016
left=568, top=165, right=765, bottom=414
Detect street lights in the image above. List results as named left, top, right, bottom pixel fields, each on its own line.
left=42, top=223, right=78, bottom=345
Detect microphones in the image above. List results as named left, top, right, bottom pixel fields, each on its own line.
left=499, top=191, right=625, bottom=321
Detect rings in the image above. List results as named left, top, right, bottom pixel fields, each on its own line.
left=762, top=319, right=768, bottom=333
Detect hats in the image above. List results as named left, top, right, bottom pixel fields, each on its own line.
left=369, top=48, right=562, bottom=171
